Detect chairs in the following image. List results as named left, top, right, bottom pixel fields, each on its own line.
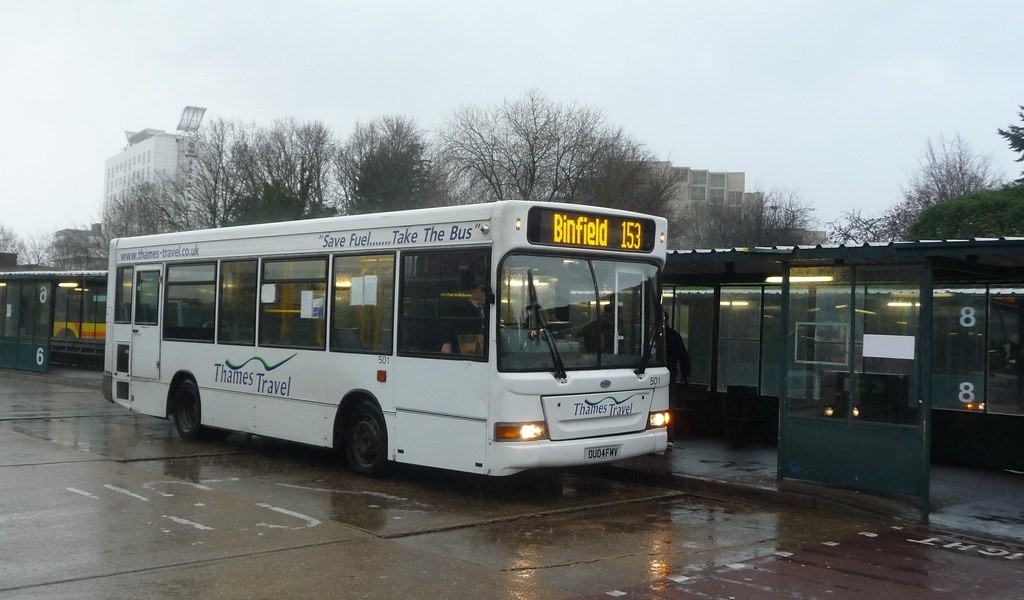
left=119, top=299, right=451, bottom=355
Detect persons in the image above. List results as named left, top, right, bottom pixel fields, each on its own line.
left=439, top=278, right=506, bottom=354
left=578, top=303, right=622, bottom=354
left=645, top=311, right=692, bottom=450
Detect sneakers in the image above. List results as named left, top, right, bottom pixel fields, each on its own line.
left=667, top=439, right=674, bottom=449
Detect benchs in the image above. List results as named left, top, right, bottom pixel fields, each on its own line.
left=672, top=383, right=708, bottom=440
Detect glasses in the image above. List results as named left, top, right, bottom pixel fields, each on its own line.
left=483, top=286, right=488, bottom=292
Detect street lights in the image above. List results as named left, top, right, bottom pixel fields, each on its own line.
left=770, top=206, right=815, bottom=229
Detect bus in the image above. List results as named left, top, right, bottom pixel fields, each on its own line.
left=101, top=199, right=671, bottom=479
left=52, top=288, right=106, bottom=338
